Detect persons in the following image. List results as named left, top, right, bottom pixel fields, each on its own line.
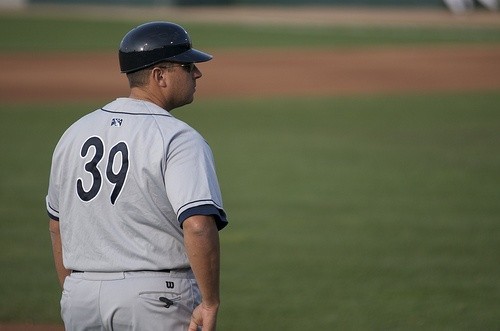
left=46, top=22, right=228, bottom=331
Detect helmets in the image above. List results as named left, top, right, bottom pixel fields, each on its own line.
left=119, top=21, right=214, bottom=73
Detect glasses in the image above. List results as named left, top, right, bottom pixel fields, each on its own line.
left=149, top=62, right=195, bottom=72
